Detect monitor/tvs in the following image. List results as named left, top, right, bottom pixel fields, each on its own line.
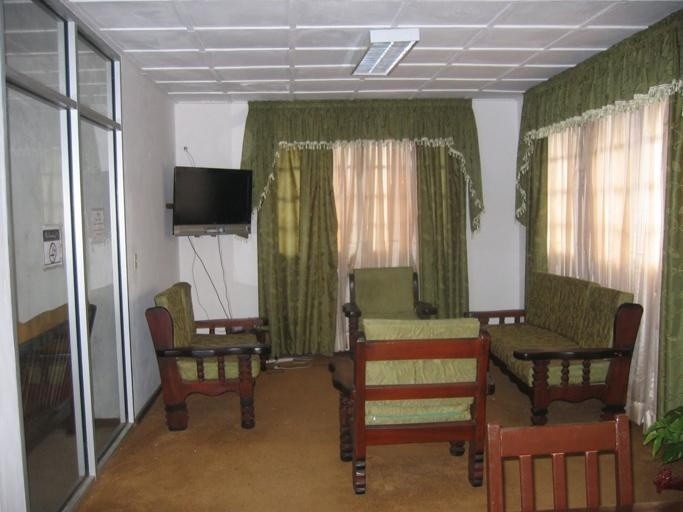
left=172, top=166, right=253, bottom=237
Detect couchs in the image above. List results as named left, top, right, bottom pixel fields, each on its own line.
left=17, top=301, right=98, bottom=457
left=146, top=282, right=271, bottom=430
left=464, top=272, right=643, bottom=426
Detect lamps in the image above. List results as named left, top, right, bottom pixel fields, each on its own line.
left=350, top=28, right=420, bottom=77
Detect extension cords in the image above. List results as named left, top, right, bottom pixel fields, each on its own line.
left=266, top=356, right=294, bottom=365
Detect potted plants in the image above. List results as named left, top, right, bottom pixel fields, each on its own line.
left=643, top=405, right=683, bottom=493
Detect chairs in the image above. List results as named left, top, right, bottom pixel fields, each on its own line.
left=342, top=266, right=438, bottom=360
left=486, top=414, right=635, bottom=511
left=329, top=317, right=496, bottom=494
